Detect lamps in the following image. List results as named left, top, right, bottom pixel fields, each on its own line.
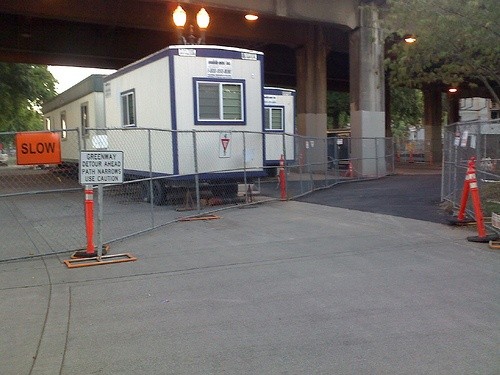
left=404, top=32, right=418, bottom=44
left=447, top=85, right=459, bottom=93
left=244, top=14, right=259, bottom=22
left=172, top=4, right=187, bottom=28
left=196, top=8, right=210, bottom=29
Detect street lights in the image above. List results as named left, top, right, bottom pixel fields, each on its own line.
left=173, top=5, right=210, bottom=44
left=383, top=32, right=418, bottom=172
left=435, top=84, right=458, bottom=168
